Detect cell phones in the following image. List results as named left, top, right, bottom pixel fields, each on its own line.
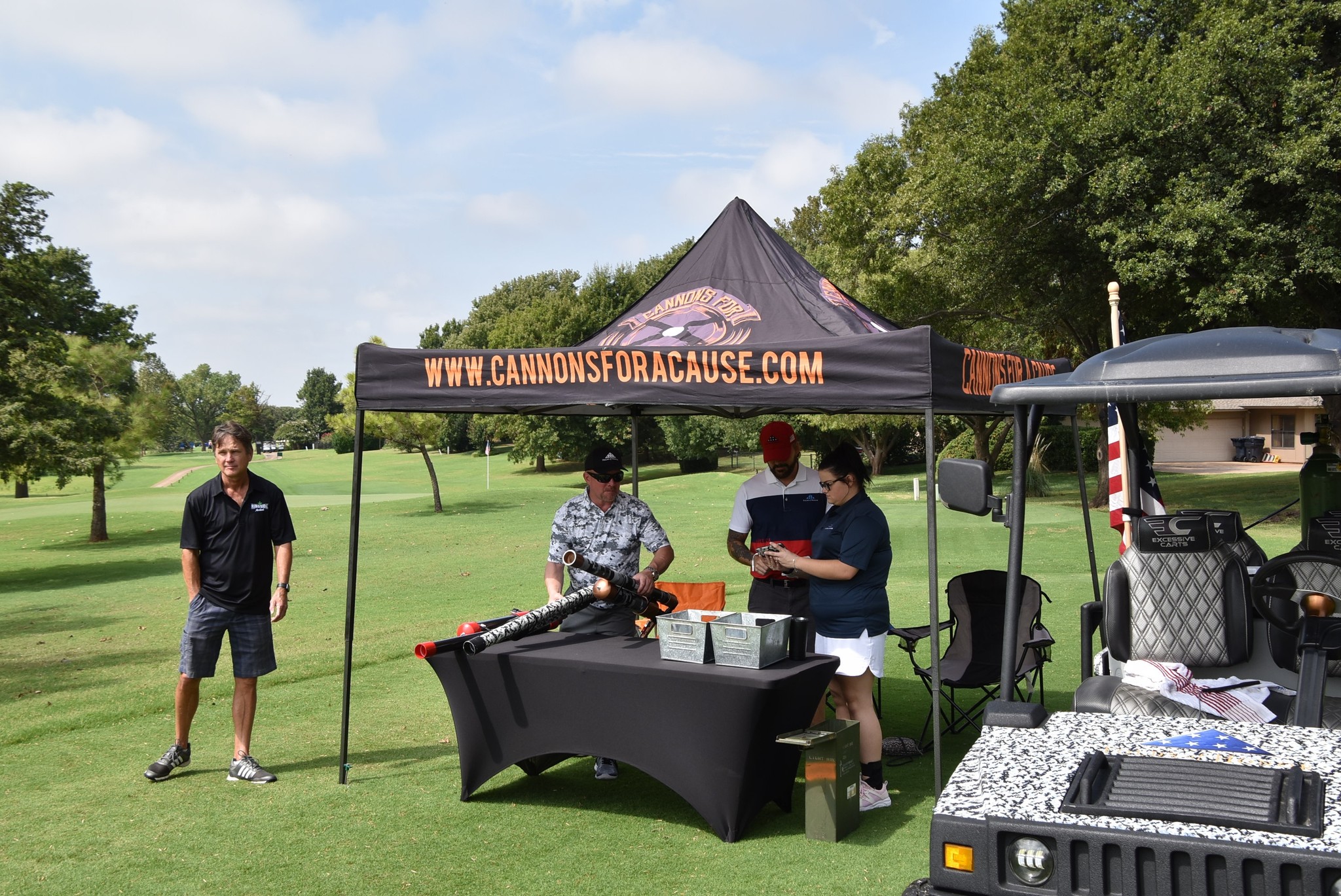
left=755, top=543, right=786, bottom=557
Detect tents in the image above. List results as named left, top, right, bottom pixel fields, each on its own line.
left=335, top=197, right=1080, bottom=802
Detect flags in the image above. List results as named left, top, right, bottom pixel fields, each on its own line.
left=1107, top=308, right=1166, bottom=557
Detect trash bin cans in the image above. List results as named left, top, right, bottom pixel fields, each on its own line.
left=1230, top=436, right=1246, bottom=462
left=1241, top=436, right=1265, bottom=462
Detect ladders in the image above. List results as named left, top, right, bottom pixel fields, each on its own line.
left=1262, top=453, right=1280, bottom=463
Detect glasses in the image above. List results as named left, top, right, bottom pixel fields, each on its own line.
left=586, top=470, right=624, bottom=483
left=819, top=474, right=854, bottom=491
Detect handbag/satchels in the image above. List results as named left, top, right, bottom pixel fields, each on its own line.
left=882, top=736, right=923, bottom=767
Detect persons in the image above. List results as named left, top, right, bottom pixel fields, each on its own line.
left=208, top=440, right=212, bottom=453
left=727, top=421, right=834, bottom=728
left=764, top=435, right=894, bottom=812
left=142, top=422, right=293, bottom=783
left=544, top=446, right=675, bottom=780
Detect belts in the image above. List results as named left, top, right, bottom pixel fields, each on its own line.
left=754, top=577, right=810, bottom=588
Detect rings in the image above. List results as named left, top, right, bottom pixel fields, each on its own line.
left=777, top=557, right=778, bottom=561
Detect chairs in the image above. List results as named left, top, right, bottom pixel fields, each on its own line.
left=890, top=570, right=1056, bottom=753
left=632, top=579, right=727, bottom=638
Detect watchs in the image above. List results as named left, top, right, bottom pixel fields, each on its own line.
left=644, top=566, right=659, bottom=581
left=277, top=583, right=290, bottom=592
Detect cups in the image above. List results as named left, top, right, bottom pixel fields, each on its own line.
left=789, top=616, right=809, bottom=661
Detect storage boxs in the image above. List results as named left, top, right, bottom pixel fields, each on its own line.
left=710, top=612, right=793, bottom=669
left=654, top=609, right=737, bottom=664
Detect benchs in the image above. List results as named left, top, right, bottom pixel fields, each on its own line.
left=1077, top=511, right=1341, bottom=729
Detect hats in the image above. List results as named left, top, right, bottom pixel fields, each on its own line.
left=585, top=444, right=628, bottom=471
left=760, top=421, right=798, bottom=463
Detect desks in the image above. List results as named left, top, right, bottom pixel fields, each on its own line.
left=425, top=628, right=839, bottom=843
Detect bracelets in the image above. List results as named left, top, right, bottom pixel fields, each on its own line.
left=792, top=554, right=800, bottom=573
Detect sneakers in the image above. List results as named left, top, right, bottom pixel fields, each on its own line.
left=226, top=756, right=277, bottom=784
left=594, top=756, right=619, bottom=780
left=145, top=743, right=191, bottom=781
left=860, top=780, right=891, bottom=811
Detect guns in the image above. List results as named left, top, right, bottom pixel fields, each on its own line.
left=563, top=548, right=681, bottom=638
left=413, top=607, right=568, bottom=660
left=462, top=582, right=598, bottom=658
